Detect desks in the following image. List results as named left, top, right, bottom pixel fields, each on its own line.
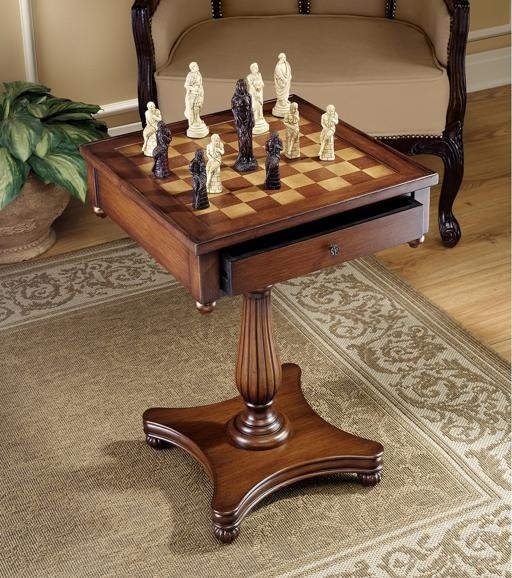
left=79, top=95, right=439, bottom=543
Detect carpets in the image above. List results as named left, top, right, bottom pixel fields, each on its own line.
left=0, top=237, right=512, bottom=578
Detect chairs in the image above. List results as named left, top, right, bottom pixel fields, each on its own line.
left=131, top=1, right=471, bottom=248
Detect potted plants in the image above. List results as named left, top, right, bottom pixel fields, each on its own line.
left=0, top=81, right=111, bottom=266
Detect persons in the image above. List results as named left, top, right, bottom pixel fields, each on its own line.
left=232, top=80, right=258, bottom=172
left=141, top=101, right=162, bottom=157
left=247, top=63, right=270, bottom=134
left=183, top=61, right=209, bottom=139
left=152, top=121, right=172, bottom=177
left=272, top=52, right=291, bottom=117
left=206, top=134, right=226, bottom=194
left=188, top=150, right=210, bottom=210
left=319, top=105, right=338, bottom=161
left=264, top=133, right=284, bottom=190
left=283, top=102, right=300, bottom=159
